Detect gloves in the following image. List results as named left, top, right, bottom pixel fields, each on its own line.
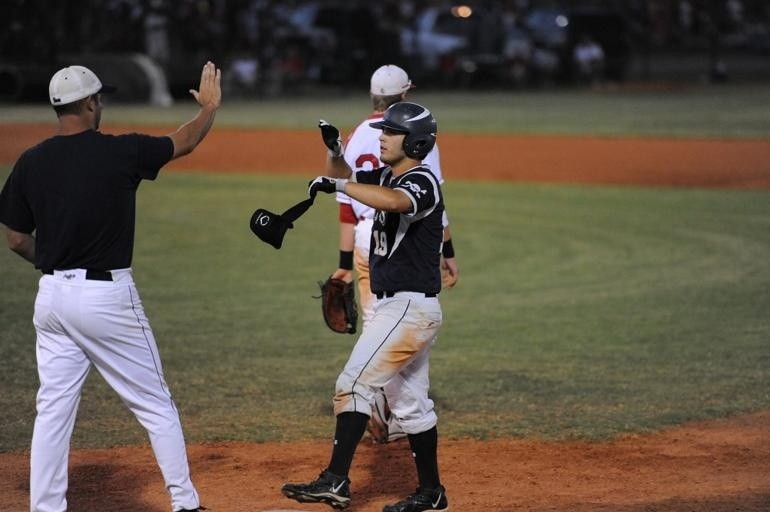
left=318, top=118, right=345, bottom=158
left=307, top=175, right=350, bottom=201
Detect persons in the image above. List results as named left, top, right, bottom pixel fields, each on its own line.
left=322, top=60, right=460, bottom=445
left=278, top=99, right=451, bottom=512
left=1, top=57, right=223, bottom=510
left=0, top=0, right=770, bottom=112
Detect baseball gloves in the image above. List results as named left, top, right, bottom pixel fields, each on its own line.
left=311, top=275, right=358, bottom=334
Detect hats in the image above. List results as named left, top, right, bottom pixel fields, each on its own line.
left=48, top=65, right=118, bottom=106
left=370, top=64, right=417, bottom=96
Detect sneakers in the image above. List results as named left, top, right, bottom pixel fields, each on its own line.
left=281, top=471, right=353, bottom=510
left=366, top=388, right=409, bottom=444
left=383, top=486, right=449, bottom=512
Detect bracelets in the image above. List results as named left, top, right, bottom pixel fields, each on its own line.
left=338, top=247, right=354, bottom=271
left=442, top=238, right=455, bottom=259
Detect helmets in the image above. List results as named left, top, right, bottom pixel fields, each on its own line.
left=369, top=102, right=437, bottom=161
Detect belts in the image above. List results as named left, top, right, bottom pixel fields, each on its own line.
left=377, top=291, right=436, bottom=300
left=43, top=269, right=113, bottom=281
